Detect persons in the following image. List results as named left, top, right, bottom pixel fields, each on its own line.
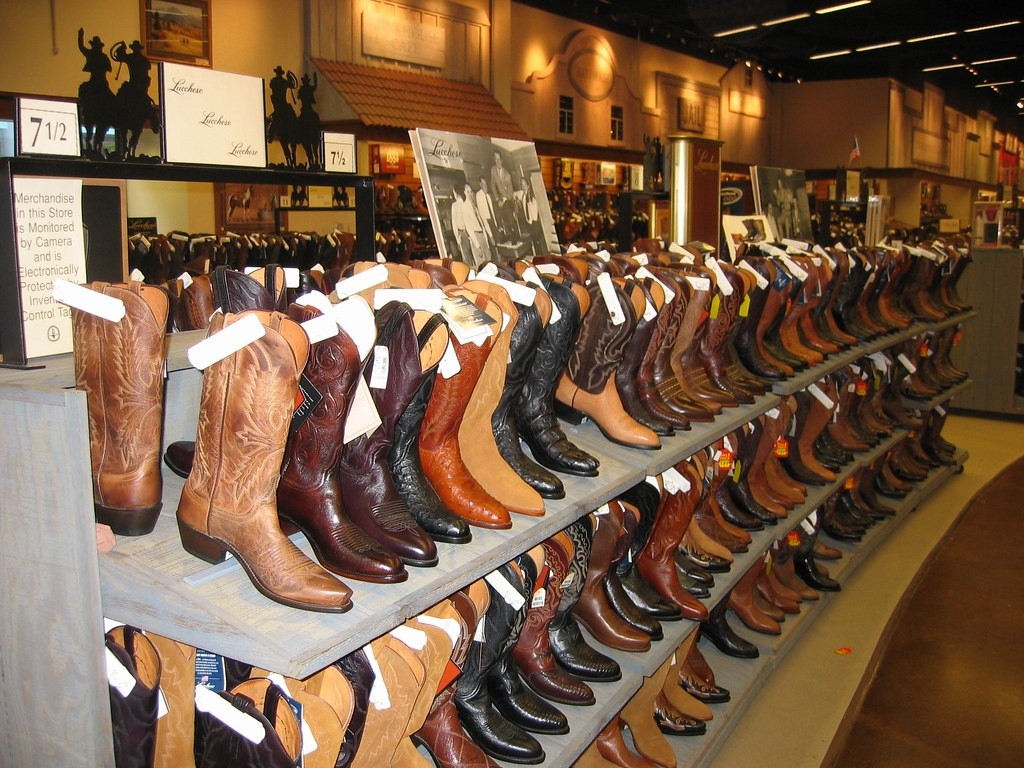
left=78, top=27, right=116, bottom=98
left=117, top=40, right=151, bottom=94
left=297, top=72, right=319, bottom=123
left=270, top=66, right=294, bottom=125
left=767, top=177, right=802, bottom=244
left=451, top=149, right=549, bottom=265
left=643, top=133, right=662, bottom=157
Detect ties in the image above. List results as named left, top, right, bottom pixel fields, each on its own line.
left=524, top=191, right=530, bottom=221
left=472, top=204, right=483, bottom=230
left=485, top=196, right=493, bottom=219
left=498, top=167, right=501, bottom=174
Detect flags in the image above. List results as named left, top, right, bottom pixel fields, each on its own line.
left=848, top=136, right=860, bottom=167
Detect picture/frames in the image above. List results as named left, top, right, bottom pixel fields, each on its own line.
left=138, top=0, right=213, bottom=69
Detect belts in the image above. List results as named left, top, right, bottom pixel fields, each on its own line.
left=487, top=219, right=493, bottom=222
left=474, top=231, right=483, bottom=233
left=458, top=229, right=464, bottom=231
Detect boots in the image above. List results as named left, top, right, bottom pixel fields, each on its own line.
left=66, top=209, right=975, bottom=768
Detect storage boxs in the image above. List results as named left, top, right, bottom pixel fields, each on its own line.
left=595, top=160, right=617, bottom=185
left=625, top=164, right=644, bottom=192
left=373, top=145, right=407, bottom=174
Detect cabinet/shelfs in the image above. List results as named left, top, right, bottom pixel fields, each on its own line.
left=0, top=156, right=976, bottom=768
left=943, top=245, right=1024, bottom=416
